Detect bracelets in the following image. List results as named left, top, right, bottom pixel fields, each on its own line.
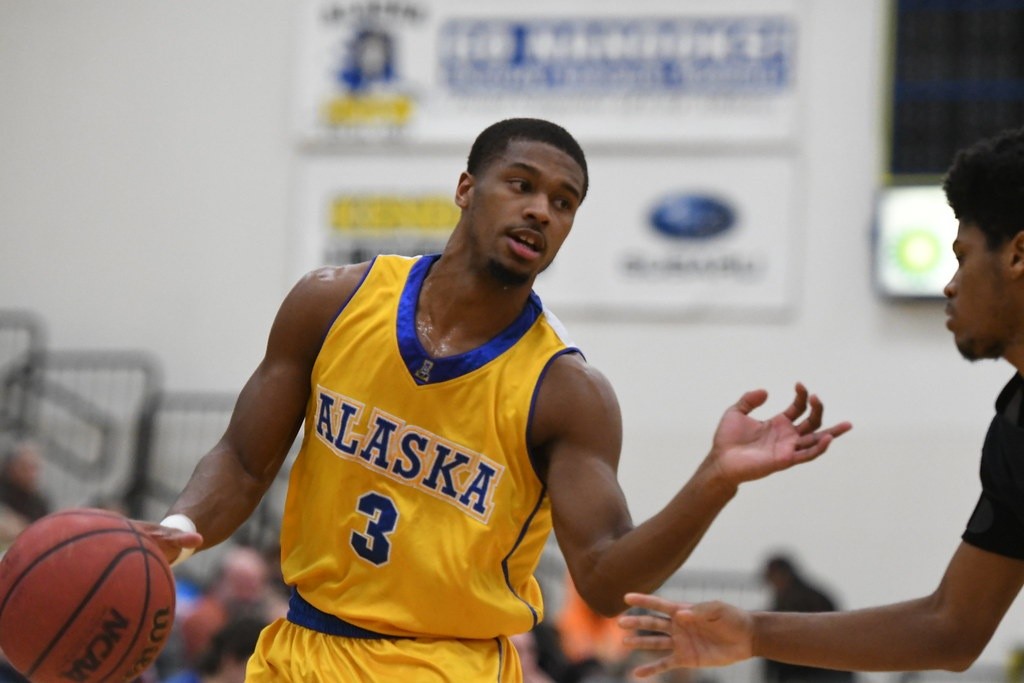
left=162, top=514, right=198, bottom=567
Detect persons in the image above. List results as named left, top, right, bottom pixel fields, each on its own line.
left=142, top=119, right=852, bottom=683
left=619, top=129, right=1024, bottom=677
left=760, top=554, right=853, bottom=682
left=182, top=552, right=288, bottom=683
left=0, top=447, right=50, bottom=534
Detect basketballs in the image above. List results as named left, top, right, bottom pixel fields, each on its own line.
left=1, top=503, right=180, bottom=683
